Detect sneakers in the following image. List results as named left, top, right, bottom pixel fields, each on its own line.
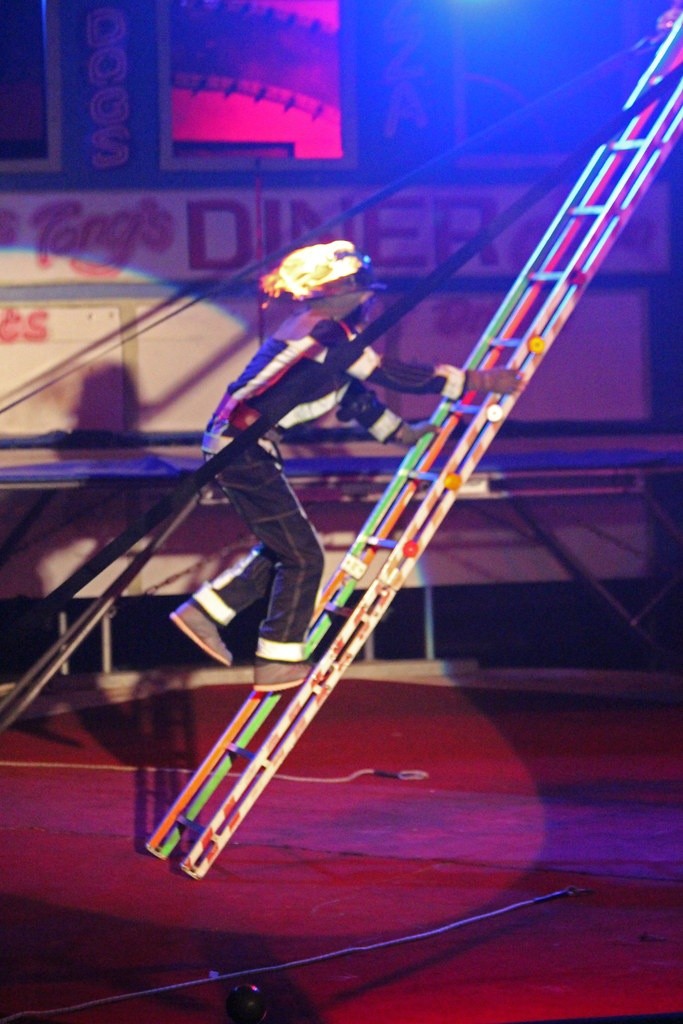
left=170, top=598, right=233, bottom=666
left=253, top=657, right=333, bottom=692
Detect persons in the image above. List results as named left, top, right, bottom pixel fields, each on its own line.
left=167, top=239, right=529, bottom=693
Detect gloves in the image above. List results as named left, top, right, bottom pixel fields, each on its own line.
left=465, top=369, right=520, bottom=394
left=396, top=421, right=439, bottom=445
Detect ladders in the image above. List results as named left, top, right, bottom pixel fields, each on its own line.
left=142, top=1, right=683, bottom=881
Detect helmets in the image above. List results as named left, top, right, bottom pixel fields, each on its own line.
left=279, top=239, right=361, bottom=295
